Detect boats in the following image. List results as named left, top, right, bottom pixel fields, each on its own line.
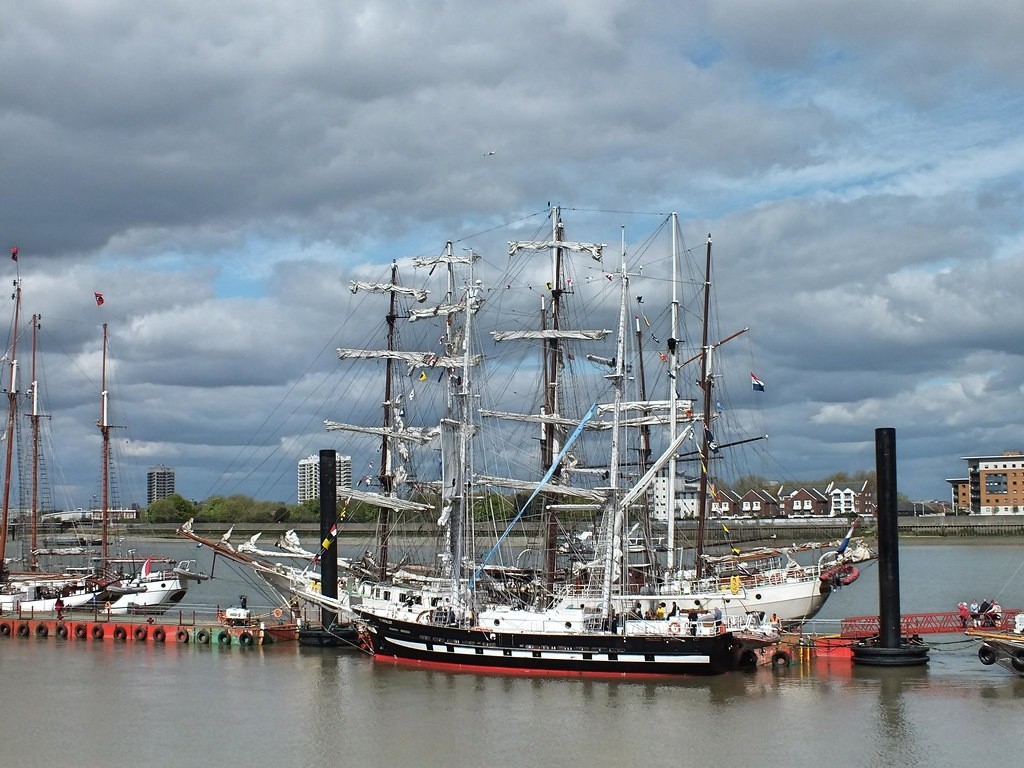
left=963, top=629, right=1024, bottom=675
left=554, top=530, right=646, bottom=553
left=41, top=537, right=86, bottom=545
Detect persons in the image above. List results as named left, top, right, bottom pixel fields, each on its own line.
left=769, top=614, right=779, bottom=622
left=970, top=600, right=981, bottom=627
left=957, top=602, right=968, bottom=628
left=241, top=596, right=247, bottom=609
left=714, top=606, right=722, bottom=632
left=978, top=599, right=1002, bottom=628
left=688, top=608, right=697, bottom=636
left=630, top=601, right=679, bottom=621
left=56, top=595, right=64, bottom=620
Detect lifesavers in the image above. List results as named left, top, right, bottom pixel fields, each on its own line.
left=75, top=625, right=86, bottom=638
left=18, top=623, right=29, bottom=636
left=105, top=602, right=110, bottom=610
left=35, top=624, right=48, bottom=637
left=239, top=632, right=252, bottom=646
left=338, top=578, right=346, bottom=590
left=669, top=622, right=681, bottom=634
left=978, top=646, right=996, bottom=665
left=176, top=629, right=189, bottom=644
left=134, top=628, right=146, bottom=641
left=153, top=628, right=165, bottom=642
left=0, top=623, right=10, bottom=636
left=771, top=651, right=790, bottom=669
left=1012, top=648, right=1024, bottom=672
left=218, top=612, right=227, bottom=624
left=56, top=624, right=68, bottom=638
left=197, top=630, right=210, bottom=644
left=793, top=570, right=802, bottom=578
left=218, top=631, right=231, bottom=645
left=92, top=626, right=103, bottom=639
left=742, top=650, right=757, bottom=667
left=114, top=627, right=126, bottom=640
left=770, top=574, right=782, bottom=585
left=274, top=608, right=282, bottom=618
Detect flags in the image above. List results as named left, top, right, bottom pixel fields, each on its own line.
left=715, top=400, right=725, bottom=415
left=751, top=372, right=763, bottom=392
left=703, top=423, right=718, bottom=454
left=697, top=447, right=740, bottom=555
left=528, top=274, right=614, bottom=291
left=356, top=443, right=383, bottom=487
left=644, top=314, right=677, bottom=379
left=140, top=557, right=152, bottom=580
left=313, top=495, right=352, bottom=571
left=400, top=334, right=445, bottom=416
left=685, top=408, right=692, bottom=417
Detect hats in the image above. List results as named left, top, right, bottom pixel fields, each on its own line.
left=982, top=598, right=987, bottom=601
left=993, top=601, right=999, bottom=604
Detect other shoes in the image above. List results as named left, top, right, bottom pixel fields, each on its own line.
left=57, top=614, right=64, bottom=620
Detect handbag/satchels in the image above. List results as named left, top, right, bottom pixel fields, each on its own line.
left=55, top=604, right=60, bottom=611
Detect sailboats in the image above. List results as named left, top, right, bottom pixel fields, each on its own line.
left=177, top=204, right=861, bottom=679
left=0, top=277, right=189, bottom=618
